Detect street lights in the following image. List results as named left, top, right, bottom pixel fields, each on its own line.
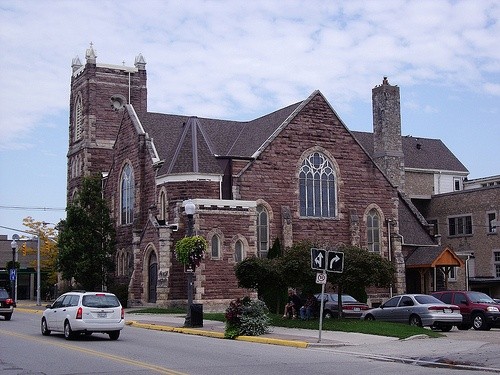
left=10, top=238, right=17, bottom=308
left=183, top=198, right=198, bottom=329
left=101, top=170, right=110, bottom=294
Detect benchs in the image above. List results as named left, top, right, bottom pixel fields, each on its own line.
left=287, top=299, right=328, bottom=320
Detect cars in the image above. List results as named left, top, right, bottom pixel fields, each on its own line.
left=360, top=294, right=462, bottom=333
left=429, top=290, right=500, bottom=331
left=299, top=292, right=368, bottom=321
left=40, top=290, right=125, bottom=341
left=0, top=288, right=15, bottom=321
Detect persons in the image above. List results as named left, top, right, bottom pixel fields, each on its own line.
left=45, top=286, right=51, bottom=302
left=281, top=285, right=302, bottom=321
left=297, top=285, right=320, bottom=321
